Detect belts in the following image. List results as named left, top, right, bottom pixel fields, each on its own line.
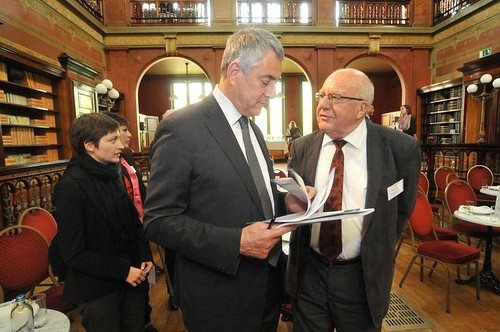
left=307, top=246, right=363, bottom=266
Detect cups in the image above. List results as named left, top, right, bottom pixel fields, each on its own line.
left=29, top=294, right=47, bottom=328
left=481, top=181, right=487, bottom=189
left=491, top=206, right=499, bottom=222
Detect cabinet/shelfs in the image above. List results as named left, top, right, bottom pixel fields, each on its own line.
left=425, top=86, right=462, bottom=143
left=0, top=51, right=65, bottom=166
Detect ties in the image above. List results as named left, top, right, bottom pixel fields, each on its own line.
left=239, top=115, right=283, bottom=267
left=319, top=140, right=347, bottom=262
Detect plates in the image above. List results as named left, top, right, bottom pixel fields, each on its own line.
left=472, top=210, right=495, bottom=214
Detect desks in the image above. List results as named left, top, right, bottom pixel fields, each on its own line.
left=452, top=210, right=500, bottom=296
left=480, top=188, right=500, bottom=210
left=32, top=308, right=71, bottom=332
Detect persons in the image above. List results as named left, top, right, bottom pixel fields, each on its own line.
left=144, top=28, right=323, bottom=332
left=285, top=120, right=303, bottom=143
left=395, top=106, right=417, bottom=139
left=283, top=68, right=421, bottom=332
left=95, top=112, right=148, bottom=225
left=51, top=112, right=161, bottom=332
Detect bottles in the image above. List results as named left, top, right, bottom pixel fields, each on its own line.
left=11, top=294, right=34, bottom=332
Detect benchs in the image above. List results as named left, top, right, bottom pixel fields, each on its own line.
left=267, top=150, right=286, bottom=161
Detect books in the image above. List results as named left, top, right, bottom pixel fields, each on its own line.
left=424, top=86, right=462, bottom=144
left=0, top=113, right=56, bottom=126
left=0, top=62, right=52, bottom=92
left=0, top=89, right=55, bottom=111
left=4, top=149, right=59, bottom=167
left=0, top=127, right=58, bottom=145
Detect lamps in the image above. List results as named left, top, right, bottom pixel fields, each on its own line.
left=466, top=84, right=479, bottom=97
left=480, top=73, right=492, bottom=92
left=492, top=78, right=500, bottom=92
left=95, top=79, right=119, bottom=107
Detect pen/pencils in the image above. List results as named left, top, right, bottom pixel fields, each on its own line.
left=268, top=214, right=278, bottom=230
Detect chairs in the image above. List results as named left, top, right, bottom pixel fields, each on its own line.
left=0, top=206, right=63, bottom=322
left=392, top=164, right=494, bottom=313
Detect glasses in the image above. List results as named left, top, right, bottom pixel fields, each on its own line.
left=314, top=92, right=368, bottom=103
